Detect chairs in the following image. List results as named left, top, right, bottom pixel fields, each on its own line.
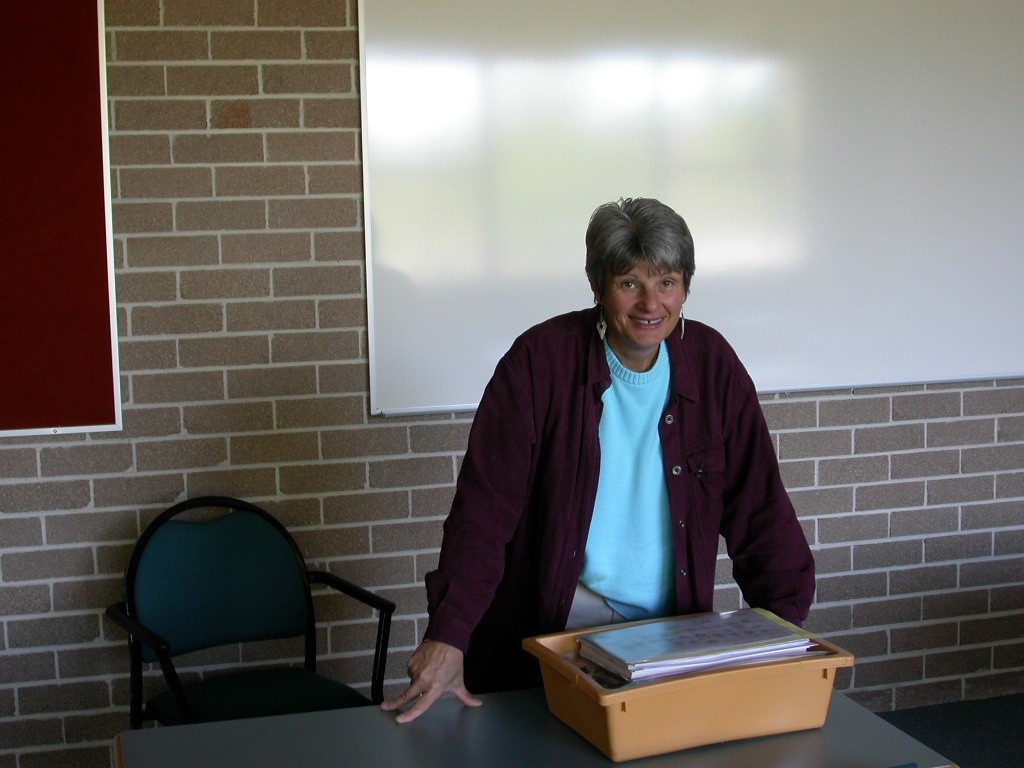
left=105, top=495, right=396, bottom=727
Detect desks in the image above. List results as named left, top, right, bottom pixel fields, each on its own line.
left=111, top=685, right=960, bottom=768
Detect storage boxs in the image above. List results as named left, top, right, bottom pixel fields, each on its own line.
left=523, top=608, right=856, bottom=762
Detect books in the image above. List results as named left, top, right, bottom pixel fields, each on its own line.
left=580, top=606, right=817, bottom=682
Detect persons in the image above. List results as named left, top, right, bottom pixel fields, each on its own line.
left=381, top=194, right=817, bottom=723
